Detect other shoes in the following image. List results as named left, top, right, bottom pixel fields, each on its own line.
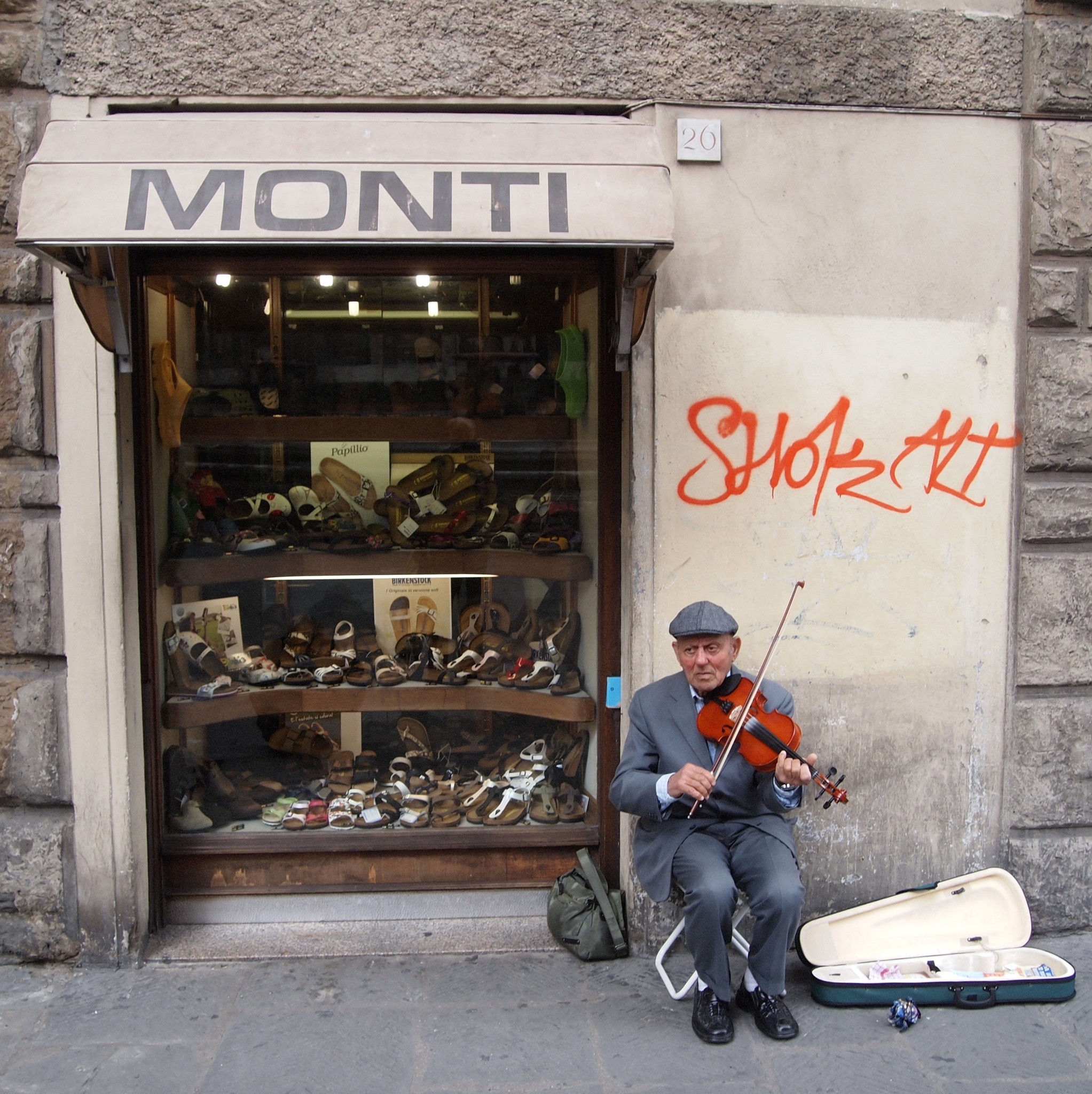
left=555, top=324, right=587, bottom=420
left=413, top=337, right=445, bottom=397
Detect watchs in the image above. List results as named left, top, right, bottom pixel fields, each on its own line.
left=773, top=778, right=797, bottom=791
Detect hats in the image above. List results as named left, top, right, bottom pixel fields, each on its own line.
left=669, top=601, right=739, bottom=640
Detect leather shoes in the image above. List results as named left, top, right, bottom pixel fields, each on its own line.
left=691, top=976, right=734, bottom=1044
left=735, top=973, right=799, bottom=1038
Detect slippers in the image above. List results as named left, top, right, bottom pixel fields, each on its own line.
left=396, top=717, right=433, bottom=769
left=258, top=360, right=280, bottom=414
left=312, top=474, right=363, bottom=531
left=163, top=727, right=590, bottom=832
left=163, top=601, right=582, bottom=698
left=389, top=596, right=411, bottom=642
left=415, top=596, right=438, bottom=636
left=152, top=338, right=192, bottom=446
left=168, top=454, right=580, bottom=556
left=319, top=458, right=377, bottom=510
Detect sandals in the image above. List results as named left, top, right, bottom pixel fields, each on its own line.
left=188, top=387, right=254, bottom=416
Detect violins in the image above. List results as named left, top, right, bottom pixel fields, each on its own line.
left=694, top=673, right=849, bottom=810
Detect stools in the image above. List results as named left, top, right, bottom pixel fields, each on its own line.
left=653, top=883, right=788, bottom=1002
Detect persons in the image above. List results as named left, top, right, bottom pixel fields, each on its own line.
left=607, top=601, right=818, bottom=1044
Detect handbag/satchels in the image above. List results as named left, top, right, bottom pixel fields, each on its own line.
left=546, top=847, right=628, bottom=961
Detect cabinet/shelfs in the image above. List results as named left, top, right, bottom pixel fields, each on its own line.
left=158, top=415, right=597, bottom=855
